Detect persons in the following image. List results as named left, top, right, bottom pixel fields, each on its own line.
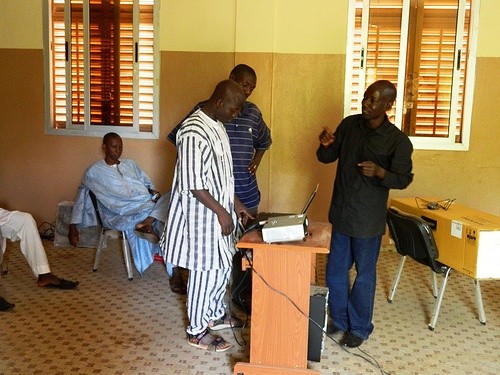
left=168, top=64, right=272, bottom=310
left=159, top=80, right=256, bottom=352
left=68, top=132, right=189, bottom=292
left=0, top=208, right=80, bottom=310
left=317, top=80, right=414, bottom=348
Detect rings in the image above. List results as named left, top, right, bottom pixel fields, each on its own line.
left=254, top=165, right=256, bottom=168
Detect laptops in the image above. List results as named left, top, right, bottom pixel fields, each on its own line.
left=251, top=184, right=319, bottom=229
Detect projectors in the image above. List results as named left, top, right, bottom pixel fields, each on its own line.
left=261, top=214, right=309, bottom=244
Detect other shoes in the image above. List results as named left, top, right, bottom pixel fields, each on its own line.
left=340, top=332, right=363, bottom=347
left=327, top=322, right=340, bottom=335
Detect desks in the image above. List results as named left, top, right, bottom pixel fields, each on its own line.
left=233, top=220, right=332, bottom=375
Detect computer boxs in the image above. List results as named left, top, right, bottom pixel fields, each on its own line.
left=308, top=286, right=329, bottom=363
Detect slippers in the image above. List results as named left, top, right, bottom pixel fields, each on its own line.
left=45, top=278, right=80, bottom=289
left=134, top=225, right=160, bottom=244
left=0, top=296, right=14, bottom=311
left=169, top=275, right=187, bottom=294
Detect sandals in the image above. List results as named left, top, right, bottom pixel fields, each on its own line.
left=208, top=312, right=249, bottom=330
left=187, top=328, right=231, bottom=352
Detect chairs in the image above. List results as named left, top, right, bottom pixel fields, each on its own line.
left=387, top=206, right=486, bottom=331
left=89, top=190, right=133, bottom=281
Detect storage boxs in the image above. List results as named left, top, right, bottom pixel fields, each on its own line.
left=389, top=196, right=500, bottom=281
